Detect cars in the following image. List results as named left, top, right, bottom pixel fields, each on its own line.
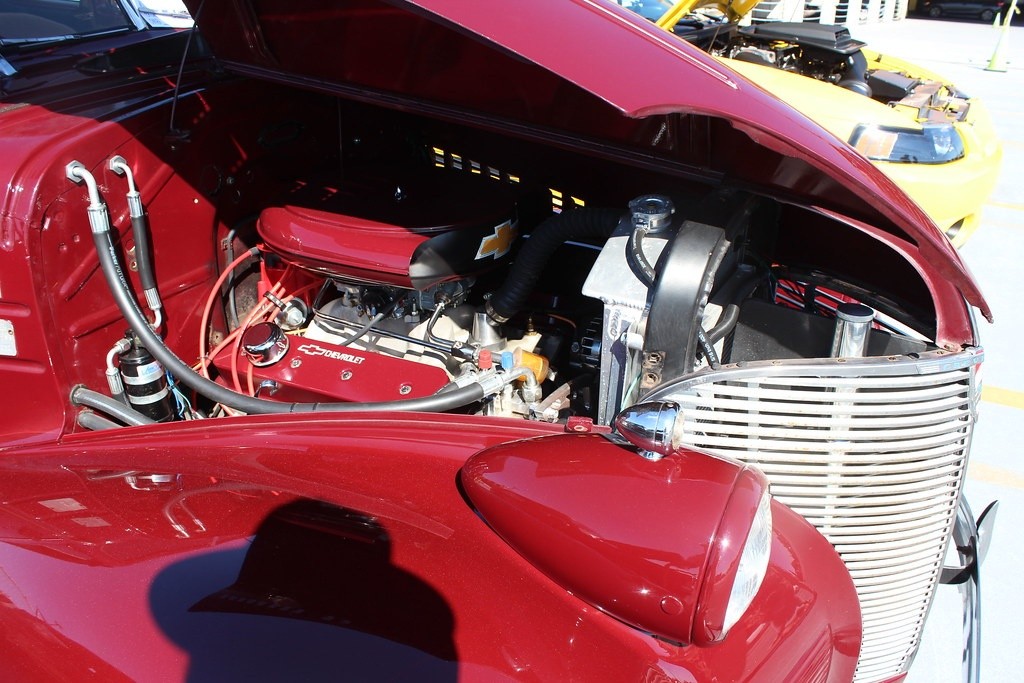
left=0, top=0, right=998, bottom=683
left=611, top=0, right=1003, bottom=251
